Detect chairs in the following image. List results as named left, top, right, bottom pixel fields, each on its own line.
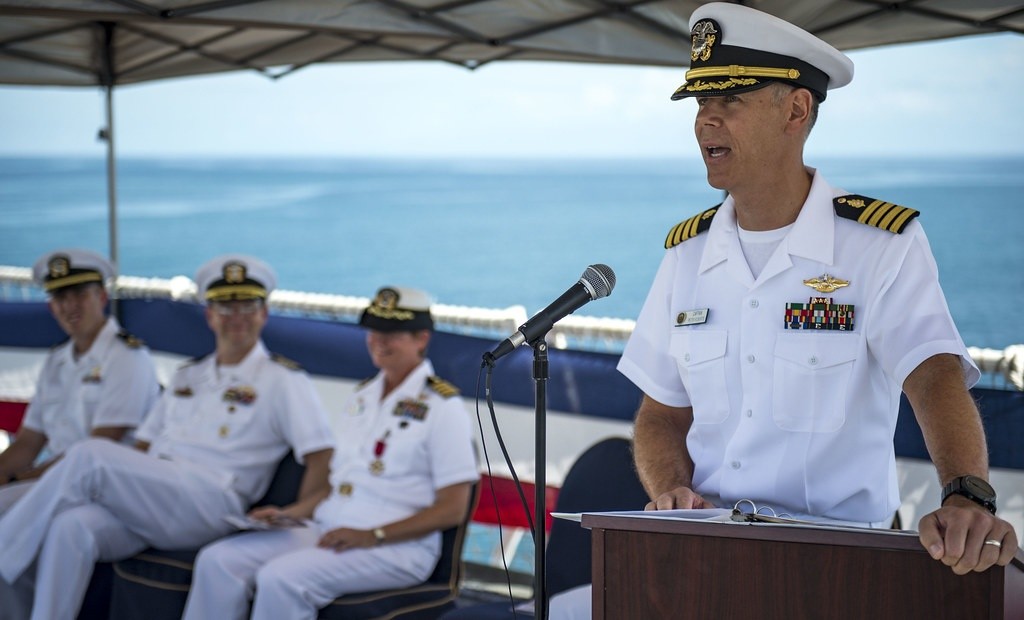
left=443, top=437, right=653, bottom=620
left=315, top=440, right=481, bottom=620
left=110, top=449, right=307, bottom=620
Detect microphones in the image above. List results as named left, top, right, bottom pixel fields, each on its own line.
left=482, top=264, right=616, bottom=365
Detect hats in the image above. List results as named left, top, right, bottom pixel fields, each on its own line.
left=671, top=2, right=855, bottom=103
left=358, top=285, right=435, bottom=333
left=34, top=251, right=118, bottom=294
left=192, top=253, right=274, bottom=304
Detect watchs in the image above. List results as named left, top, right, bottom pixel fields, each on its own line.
left=940, top=475, right=997, bottom=516
left=370, top=525, right=386, bottom=548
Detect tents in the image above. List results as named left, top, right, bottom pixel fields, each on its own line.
left=0, top=1, right=1024, bottom=293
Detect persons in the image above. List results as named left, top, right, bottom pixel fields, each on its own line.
left=616, top=2, right=1019, bottom=575
left=0, top=252, right=163, bottom=520
left=179, top=281, right=480, bottom=620
left=1, top=254, right=337, bottom=620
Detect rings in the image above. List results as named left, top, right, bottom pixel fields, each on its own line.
left=983, top=538, right=1003, bottom=547
